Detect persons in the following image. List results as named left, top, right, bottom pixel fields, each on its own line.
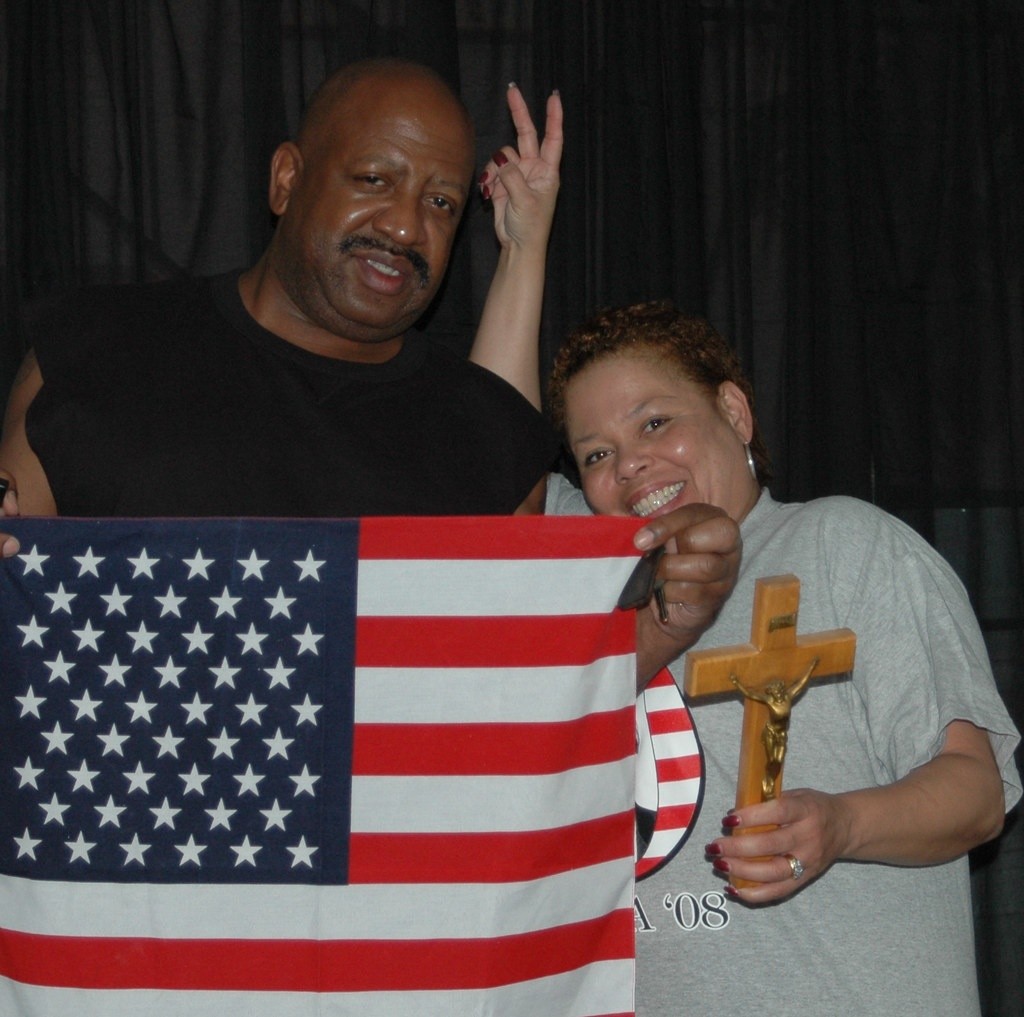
left=0, top=55, right=746, bottom=706
left=468, top=81, right=1023, bottom=1016
left=729, top=656, right=819, bottom=802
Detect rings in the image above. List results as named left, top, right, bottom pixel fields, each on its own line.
left=779, top=850, right=803, bottom=879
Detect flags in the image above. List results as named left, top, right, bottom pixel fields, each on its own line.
left=0, top=515, right=663, bottom=1016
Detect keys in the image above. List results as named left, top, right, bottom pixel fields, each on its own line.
left=619, top=545, right=672, bottom=625
